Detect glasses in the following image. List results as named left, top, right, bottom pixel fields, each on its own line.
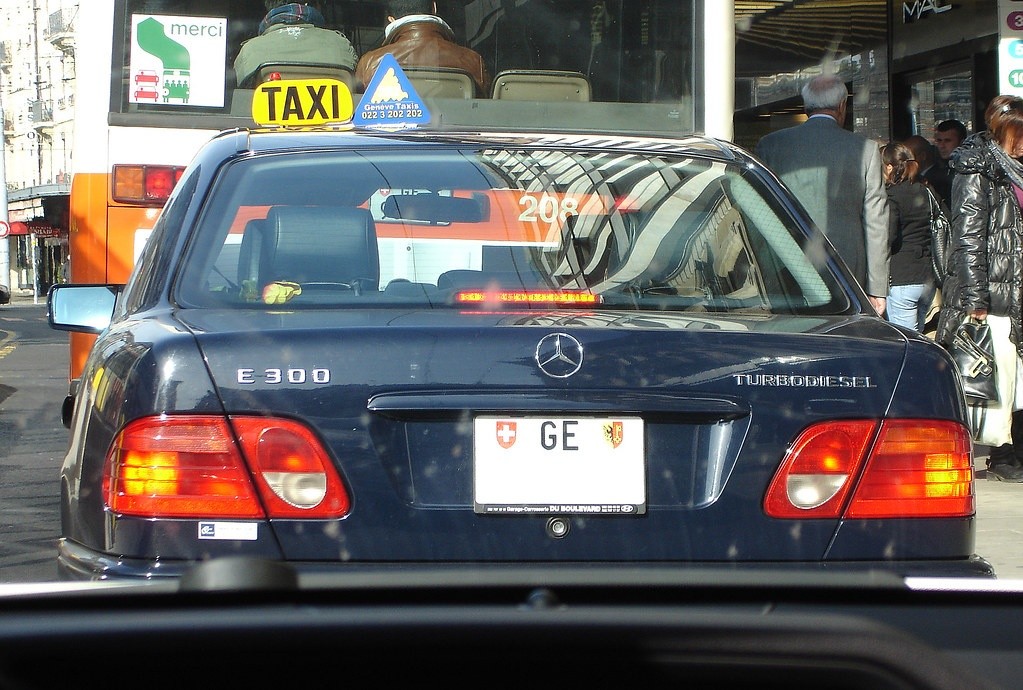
left=1001, top=101, right=1023, bottom=116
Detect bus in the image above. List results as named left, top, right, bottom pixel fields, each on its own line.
left=62, top=3, right=737, bottom=427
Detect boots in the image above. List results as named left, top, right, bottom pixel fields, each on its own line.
left=986, top=444, right=1023, bottom=482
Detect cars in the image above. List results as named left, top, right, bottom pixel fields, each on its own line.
left=45, top=73, right=993, bottom=585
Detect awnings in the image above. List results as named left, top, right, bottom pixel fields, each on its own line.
left=8, top=221, right=61, bottom=305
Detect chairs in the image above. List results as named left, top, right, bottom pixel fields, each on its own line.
left=252, top=61, right=358, bottom=94
left=257, top=205, right=381, bottom=296
left=226, top=218, right=265, bottom=291
left=489, top=71, right=593, bottom=103
left=400, top=65, right=476, bottom=99
left=558, top=213, right=644, bottom=307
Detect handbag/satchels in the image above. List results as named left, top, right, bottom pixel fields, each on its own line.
left=947, top=315, right=1023, bottom=447
left=926, top=188, right=952, bottom=281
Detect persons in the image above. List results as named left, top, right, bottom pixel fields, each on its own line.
left=879, top=144, right=948, bottom=333
left=61, top=254, right=70, bottom=283
left=232, top=0, right=360, bottom=88
left=905, top=119, right=968, bottom=211
left=355, top=0, right=492, bottom=94
left=934, top=95, right=1023, bottom=485
left=756, top=77, right=889, bottom=315
